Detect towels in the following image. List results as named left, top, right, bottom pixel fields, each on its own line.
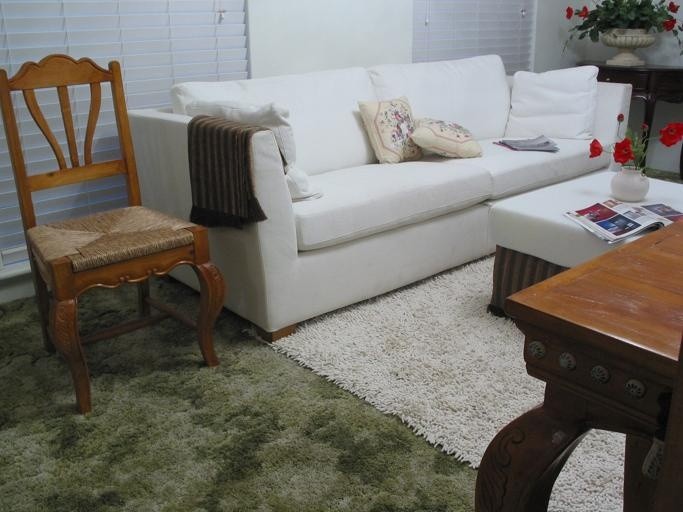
left=187, top=113, right=288, bottom=233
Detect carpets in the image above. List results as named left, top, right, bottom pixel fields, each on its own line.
left=225, top=242, right=629, bottom=509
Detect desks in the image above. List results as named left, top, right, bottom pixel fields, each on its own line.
left=471, top=215, right=683, bottom=512
left=575, top=65, right=682, bottom=184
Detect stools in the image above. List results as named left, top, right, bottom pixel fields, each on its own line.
left=484, top=171, right=682, bottom=325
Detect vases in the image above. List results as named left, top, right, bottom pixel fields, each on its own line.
left=609, top=164, right=650, bottom=206
left=593, top=29, right=662, bottom=69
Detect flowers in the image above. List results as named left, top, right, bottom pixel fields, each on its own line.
left=581, top=109, right=682, bottom=169
left=556, top=0, right=683, bottom=55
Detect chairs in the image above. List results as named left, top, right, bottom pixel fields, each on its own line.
left=0, top=54, right=233, bottom=417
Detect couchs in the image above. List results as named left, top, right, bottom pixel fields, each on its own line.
left=116, top=48, right=639, bottom=346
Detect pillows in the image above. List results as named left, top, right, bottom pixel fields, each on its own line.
left=183, top=99, right=321, bottom=201
left=501, top=64, right=599, bottom=141
left=407, top=113, right=484, bottom=162
left=356, top=94, right=421, bottom=165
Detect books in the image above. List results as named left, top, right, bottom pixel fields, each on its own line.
left=562, top=197, right=683, bottom=246
left=490, top=134, right=560, bottom=153
